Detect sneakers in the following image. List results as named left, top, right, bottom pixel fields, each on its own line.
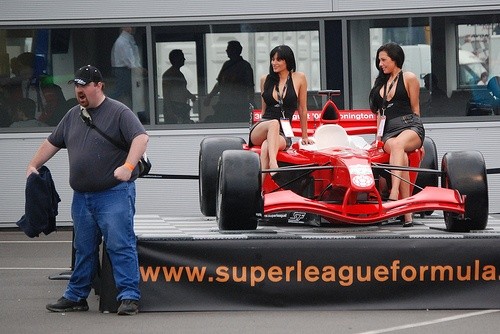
left=46, top=295, right=89, bottom=312
left=118, top=299, right=139, bottom=315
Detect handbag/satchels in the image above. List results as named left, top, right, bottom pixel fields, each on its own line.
left=138, top=152, right=152, bottom=177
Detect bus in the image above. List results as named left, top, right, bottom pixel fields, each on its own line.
left=0, top=1, right=500, bottom=229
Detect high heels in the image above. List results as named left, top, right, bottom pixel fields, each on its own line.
left=403, top=212, right=415, bottom=227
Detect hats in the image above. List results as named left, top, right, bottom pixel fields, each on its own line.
left=67, top=65, right=103, bottom=86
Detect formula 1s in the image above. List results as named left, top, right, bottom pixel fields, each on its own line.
left=197, top=89, right=500, bottom=233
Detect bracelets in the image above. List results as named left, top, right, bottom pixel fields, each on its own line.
left=124, top=162, right=135, bottom=171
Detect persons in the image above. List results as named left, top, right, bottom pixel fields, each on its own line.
left=477, top=72, right=489, bottom=86
left=423, top=73, right=449, bottom=103
left=2, top=25, right=148, bottom=124
left=203, top=39, right=255, bottom=122
left=162, top=49, right=197, bottom=124
left=369, top=42, right=426, bottom=226
left=28, top=64, right=150, bottom=316
left=249, top=45, right=314, bottom=178
